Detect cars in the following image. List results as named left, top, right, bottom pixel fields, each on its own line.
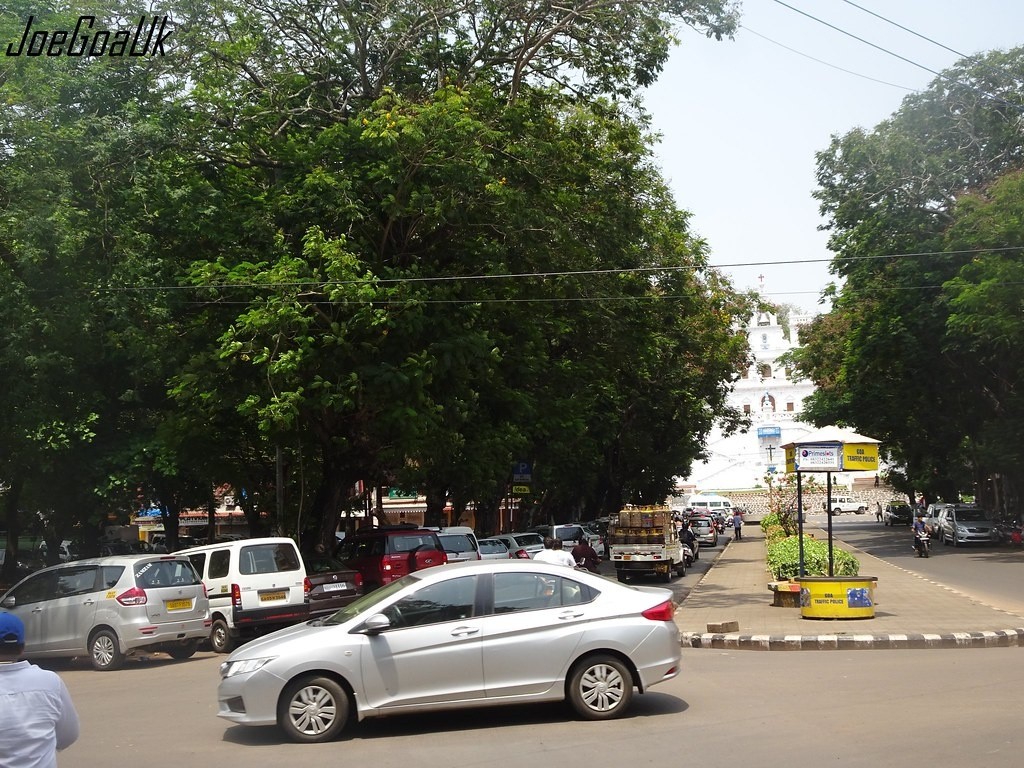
left=682, top=508, right=693, bottom=518
left=215, top=558, right=683, bottom=743
left=576, top=515, right=613, bottom=559
left=691, top=507, right=739, bottom=528
left=0, top=553, right=213, bottom=673
left=420, top=527, right=481, bottom=565
left=0, top=533, right=246, bottom=600
left=686, top=515, right=718, bottom=548
left=296, top=557, right=365, bottom=627
left=486, top=532, right=548, bottom=560
left=476, top=539, right=513, bottom=562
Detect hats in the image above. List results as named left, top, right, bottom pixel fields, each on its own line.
left=0, top=611, right=25, bottom=645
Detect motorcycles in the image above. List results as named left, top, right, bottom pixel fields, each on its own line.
left=911, top=529, right=932, bottom=558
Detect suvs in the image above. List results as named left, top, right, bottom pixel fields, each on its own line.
left=912, top=502, right=971, bottom=538
left=936, top=503, right=1003, bottom=546
left=823, top=495, right=870, bottom=515
left=332, top=523, right=449, bottom=596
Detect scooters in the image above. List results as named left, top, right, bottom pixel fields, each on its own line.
left=716, top=520, right=725, bottom=534
left=681, top=533, right=702, bottom=568
left=990, top=518, right=1024, bottom=548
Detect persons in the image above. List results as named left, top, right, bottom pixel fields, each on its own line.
left=911, top=515, right=932, bottom=551
left=674, top=509, right=742, bottom=554
left=0, top=611, right=79, bottom=768
left=874, top=473, right=879, bottom=487
left=875, top=501, right=883, bottom=523
left=533, top=536, right=602, bottom=575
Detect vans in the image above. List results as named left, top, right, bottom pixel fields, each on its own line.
left=526, top=523, right=588, bottom=554
left=148, top=536, right=312, bottom=650
left=689, top=499, right=733, bottom=512
left=884, top=503, right=914, bottom=526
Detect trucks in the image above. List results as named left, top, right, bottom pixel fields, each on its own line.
left=609, top=516, right=687, bottom=582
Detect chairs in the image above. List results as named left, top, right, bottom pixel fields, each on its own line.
left=546, top=587, right=581, bottom=609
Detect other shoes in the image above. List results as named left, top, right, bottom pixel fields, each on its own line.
left=915, top=547, right=918, bottom=552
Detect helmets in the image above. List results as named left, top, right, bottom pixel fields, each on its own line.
left=682, top=521, right=689, bottom=529
